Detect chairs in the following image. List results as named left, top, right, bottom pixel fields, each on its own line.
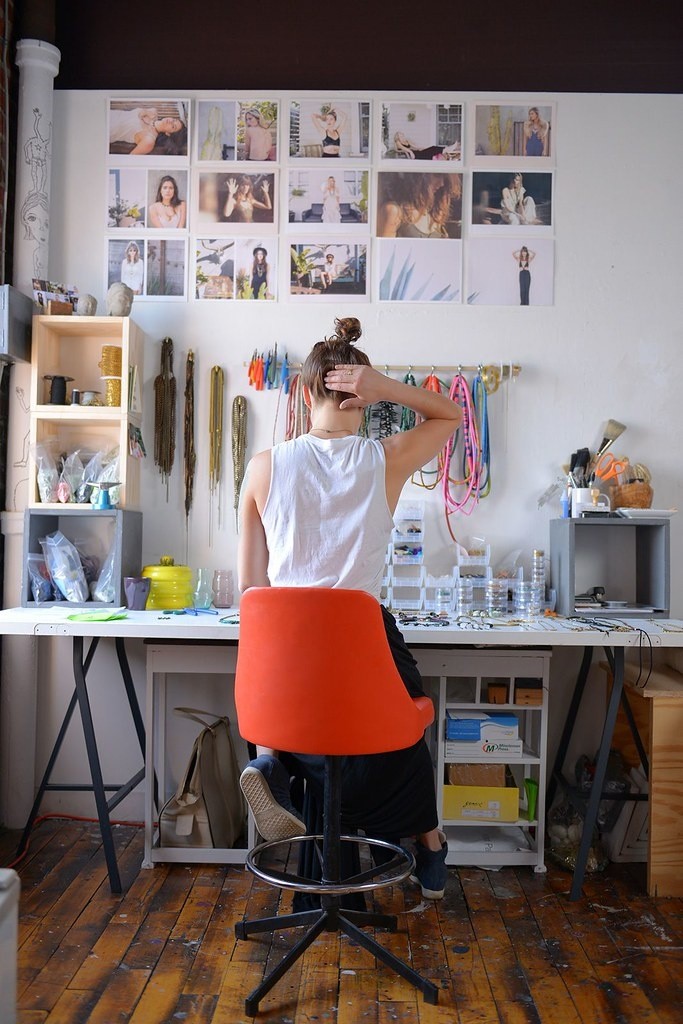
left=231, top=586, right=440, bottom=1016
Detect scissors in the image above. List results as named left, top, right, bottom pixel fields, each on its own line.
left=592, top=453, right=626, bottom=489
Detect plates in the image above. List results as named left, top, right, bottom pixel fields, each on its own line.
left=615, top=509, right=678, bottom=520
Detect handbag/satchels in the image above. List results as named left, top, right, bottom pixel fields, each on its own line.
left=159, top=706, right=248, bottom=849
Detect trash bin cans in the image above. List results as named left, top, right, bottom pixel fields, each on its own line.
left=0, top=866, right=22, bottom=1024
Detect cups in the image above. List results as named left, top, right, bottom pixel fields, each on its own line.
left=194, top=568, right=215, bottom=610
left=211, top=569, right=233, bottom=609
left=123, top=576, right=151, bottom=611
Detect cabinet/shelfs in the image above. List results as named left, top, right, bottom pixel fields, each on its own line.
left=0, top=603, right=683, bottom=907
left=28, top=313, right=144, bottom=512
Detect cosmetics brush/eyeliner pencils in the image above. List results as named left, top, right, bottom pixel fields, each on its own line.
left=565, top=419, right=628, bottom=488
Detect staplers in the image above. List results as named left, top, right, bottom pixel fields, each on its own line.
left=586, top=586, right=609, bottom=607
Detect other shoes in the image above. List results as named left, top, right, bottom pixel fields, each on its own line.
left=239, top=754, right=307, bottom=842
left=408, top=832, right=448, bottom=900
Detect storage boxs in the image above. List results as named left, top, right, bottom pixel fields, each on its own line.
left=442, top=763, right=520, bottom=822
left=444, top=711, right=523, bottom=758
left=514, top=686, right=544, bottom=706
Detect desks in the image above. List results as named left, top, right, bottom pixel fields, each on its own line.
left=140, top=637, right=553, bottom=877
left=600, top=659, right=683, bottom=904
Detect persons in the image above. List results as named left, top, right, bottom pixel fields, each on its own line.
left=129, top=433, right=143, bottom=455
left=33, top=281, right=77, bottom=311
left=78, top=283, right=133, bottom=317
left=238, top=317, right=463, bottom=901
left=100, top=107, right=549, bottom=313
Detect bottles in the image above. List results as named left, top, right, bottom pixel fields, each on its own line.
left=559, top=489, right=569, bottom=518
left=435, top=575, right=452, bottom=613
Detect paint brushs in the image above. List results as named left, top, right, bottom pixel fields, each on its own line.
left=565, top=418, right=626, bottom=515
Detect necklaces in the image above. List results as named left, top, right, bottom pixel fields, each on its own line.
left=307, top=423, right=352, bottom=438
left=390, top=607, right=683, bottom=689
left=149, top=117, right=158, bottom=130
left=256, top=265, right=264, bottom=278
left=141, top=113, right=155, bottom=127
left=160, top=201, right=170, bottom=207
